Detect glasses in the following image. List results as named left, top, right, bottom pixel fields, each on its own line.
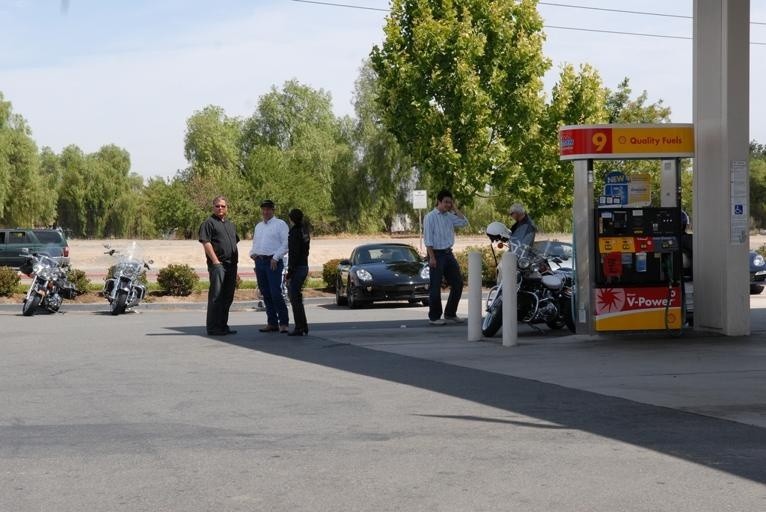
left=215, top=206, right=225, bottom=207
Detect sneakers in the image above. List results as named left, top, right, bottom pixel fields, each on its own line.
left=429, top=319, right=445, bottom=325
left=445, top=315, right=464, bottom=323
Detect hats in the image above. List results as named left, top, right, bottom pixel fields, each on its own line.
left=260, top=200, right=273, bottom=207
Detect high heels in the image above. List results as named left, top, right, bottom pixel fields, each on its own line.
left=288, top=327, right=308, bottom=336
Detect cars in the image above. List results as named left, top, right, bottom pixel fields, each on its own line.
left=749, top=249, right=766, bottom=297
left=496, top=240, right=575, bottom=280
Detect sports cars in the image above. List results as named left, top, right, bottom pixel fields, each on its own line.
left=333, top=238, right=430, bottom=309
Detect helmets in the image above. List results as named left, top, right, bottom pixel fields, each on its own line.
left=486, top=222, right=512, bottom=241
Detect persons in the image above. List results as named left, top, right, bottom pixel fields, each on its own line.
left=249, top=200, right=289, bottom=332
left=423, top=189, right=469, bottom=325
left=510, top=203, right=535, bottom=257
left=284, top=209, right=310, bottom=336
left=199, top=196, right=240, bottom=335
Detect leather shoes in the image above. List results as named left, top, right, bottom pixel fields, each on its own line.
left=259, top=324, right=288, bottom=333
left=208, top=329, right=237, bottom=335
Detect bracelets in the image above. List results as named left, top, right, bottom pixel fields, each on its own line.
left=456, top=210, right=459, bottom=213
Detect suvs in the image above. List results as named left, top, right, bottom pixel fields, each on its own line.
left=0, top=227, right=70, bottom=278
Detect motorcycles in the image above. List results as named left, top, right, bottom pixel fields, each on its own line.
left=99, top=240, right=153, bottom=315
left=254, top=255, right=290, bottom=309
left=13, top=246, right=79, bottom=316
left=481, top=221, right=575, bottom=339
